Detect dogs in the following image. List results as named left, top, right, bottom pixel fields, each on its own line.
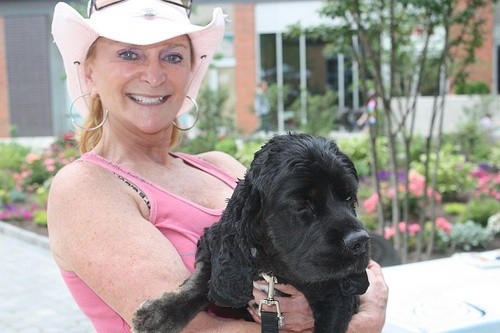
left=132, top=133, right=371, bottom=333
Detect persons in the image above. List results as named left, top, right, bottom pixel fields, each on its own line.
left=482, top=112, right=493, bottom=133
left=253, top=81, right=271, bottom=135
left=357, top=89, right=380, bottom=137
left=47, top=0, right=389, bottom=333
